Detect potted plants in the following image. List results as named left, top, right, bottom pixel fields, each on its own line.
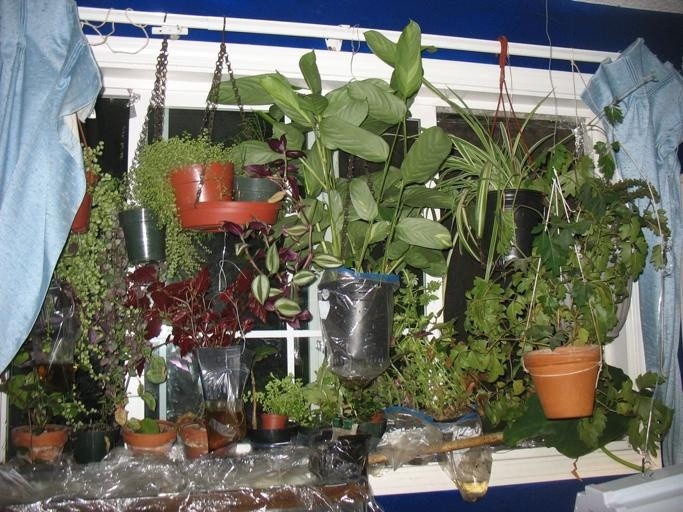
left=414, top=69, right=576, bottom=268
left=55, top=113, right=128, bottom=390
left=73, top=393, right=121, bottom=465
left=1, top=351, right=77, bottom=462
left=236, top=133, right=298, bottom=202
left=462, top=157, right=673, bottom=458
left=206, top=18, right=459, bottom=383
left=242, top=371, right=314, bottom=430
left=129, top=125, right=235, bottom=283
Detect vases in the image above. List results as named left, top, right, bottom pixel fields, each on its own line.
left=117, top=205, right=167, bottom=263
left=181, top=425, right=209, bottom=461
left=120, top=420, right=178, bottom=458
